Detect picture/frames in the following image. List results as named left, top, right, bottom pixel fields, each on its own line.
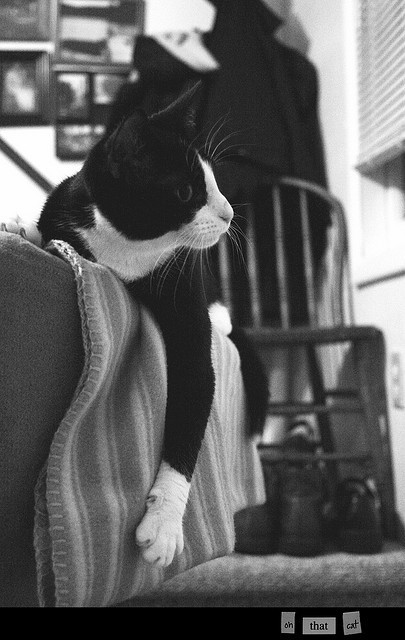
left=0, top=49, right=55, bottom=127
left=50, top=62, right=134, bottom=162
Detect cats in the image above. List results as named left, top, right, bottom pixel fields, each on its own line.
left=37, top=80, right=235, bottom=567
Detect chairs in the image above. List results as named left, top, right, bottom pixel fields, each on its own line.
left=220, top=174, right=394, bottom=535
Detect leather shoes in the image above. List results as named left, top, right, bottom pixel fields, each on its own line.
left=234, top=470, right=277, bottom=556
left=280, top=471, right=332, bottom=557
left=322, top=476, right=381, bottom=552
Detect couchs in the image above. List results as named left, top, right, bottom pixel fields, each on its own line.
left=0, top=230, right=265, bottom=609
